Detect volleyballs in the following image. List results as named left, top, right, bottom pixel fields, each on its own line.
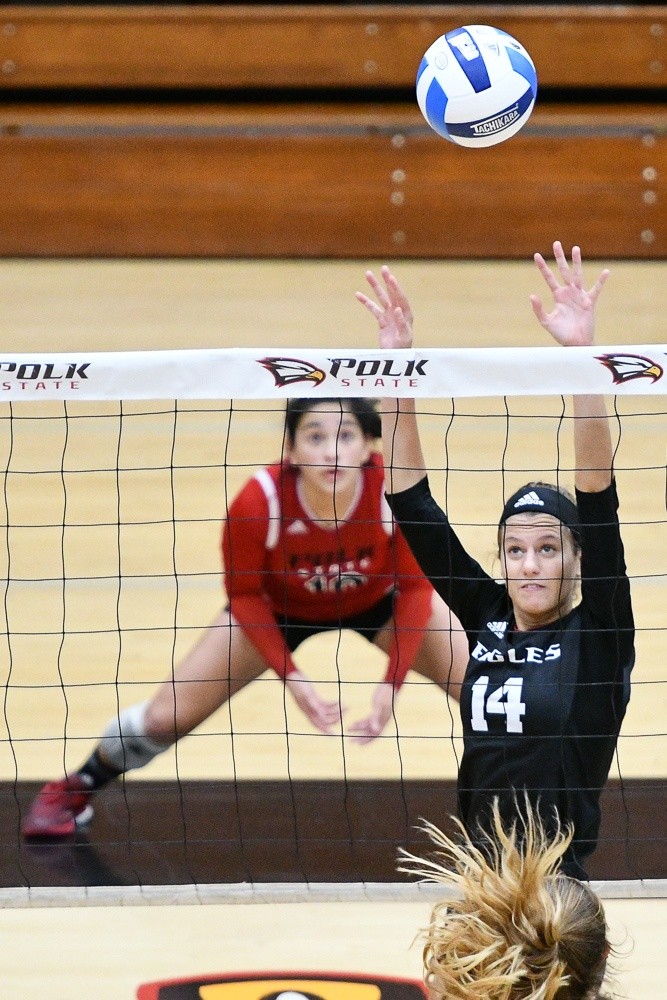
left=416, top=24, right=537, bottom=148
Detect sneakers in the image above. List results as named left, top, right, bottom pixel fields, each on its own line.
left=21, top=773, right=93, bottom=838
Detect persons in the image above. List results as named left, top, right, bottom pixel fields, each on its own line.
left=353, top=242, right=637, bottom=1000
left=17, top=397, right=468, bottom=843
